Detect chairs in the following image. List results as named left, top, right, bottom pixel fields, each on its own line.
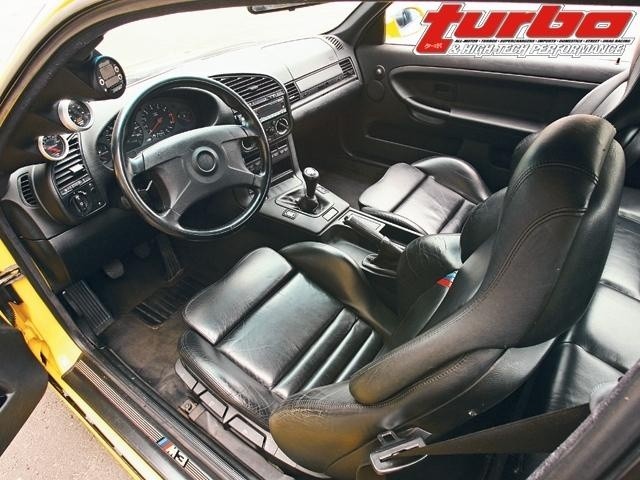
left=359, top=38, right=640, bottom=268
left=179, top=114, right=626, bottom=480
